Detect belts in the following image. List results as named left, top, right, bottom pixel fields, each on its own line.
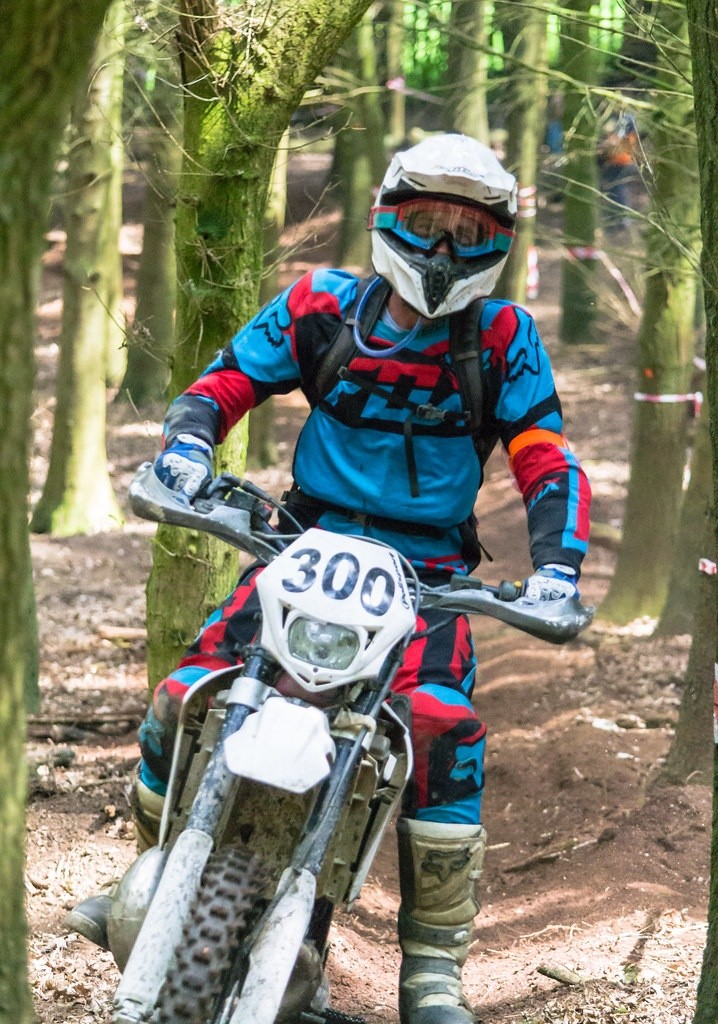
left=320, top=502, right=448, bottom=539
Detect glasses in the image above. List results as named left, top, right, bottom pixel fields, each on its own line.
left=387, top=198, right=503, bottom=258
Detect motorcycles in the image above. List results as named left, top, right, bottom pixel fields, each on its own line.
left=104, top=463, right=595, bottom=1024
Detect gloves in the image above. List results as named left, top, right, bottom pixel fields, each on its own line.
left=526, top=563, right=579, bottom=602
left=155, top=433, right=214, bottom=505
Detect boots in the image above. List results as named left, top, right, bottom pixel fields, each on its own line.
left=65, top=760, right=170, bottom=950
left=396, top=816, right=488, bottom=1024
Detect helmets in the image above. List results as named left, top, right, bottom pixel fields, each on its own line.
left=372, top=134, right=520, bottom=318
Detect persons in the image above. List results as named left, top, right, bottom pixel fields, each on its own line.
left=533, top=90, right=653, bottom=227
left=67, top=133, right=589, bottom=1024
